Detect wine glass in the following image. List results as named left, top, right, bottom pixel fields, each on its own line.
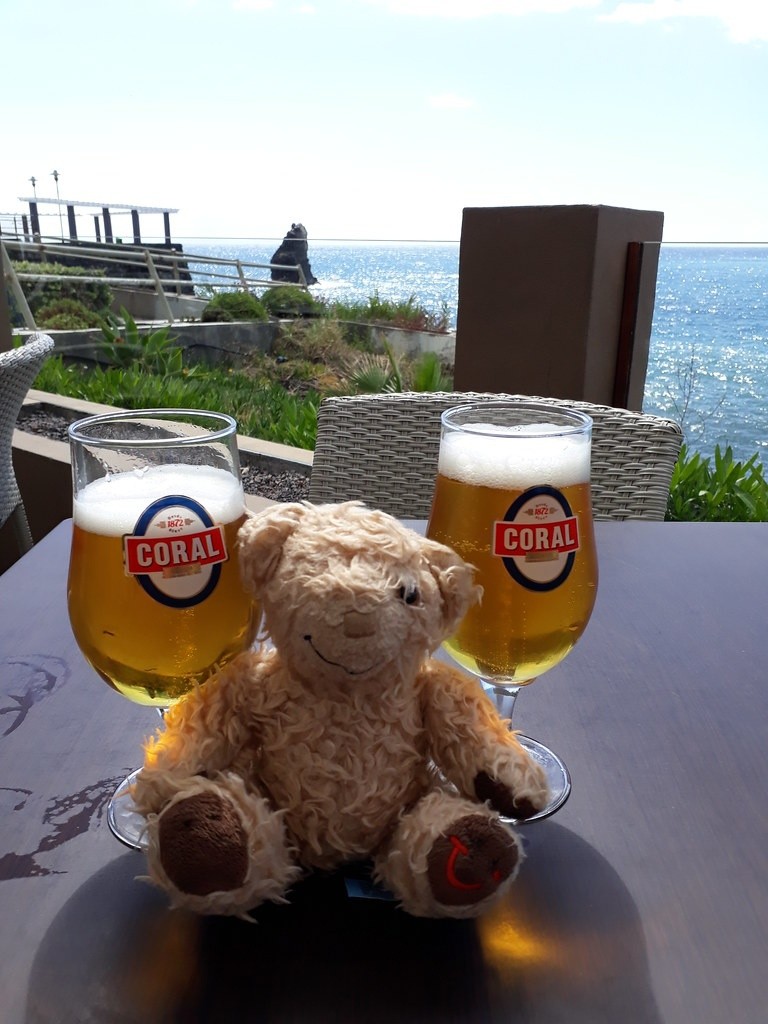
left=422, top=402, right=601, bottom=825
left=65, top=407, right=260, bottom=851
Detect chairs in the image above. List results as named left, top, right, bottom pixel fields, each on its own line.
left=307, top=390, right=686, bottom=519
left=0, top=332, right=55, bottom=557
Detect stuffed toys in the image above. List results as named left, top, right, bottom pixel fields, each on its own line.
left=132, top=499, right=549, bottom=921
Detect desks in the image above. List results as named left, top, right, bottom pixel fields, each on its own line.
left=0, top=519, right=768, bottom=1024
